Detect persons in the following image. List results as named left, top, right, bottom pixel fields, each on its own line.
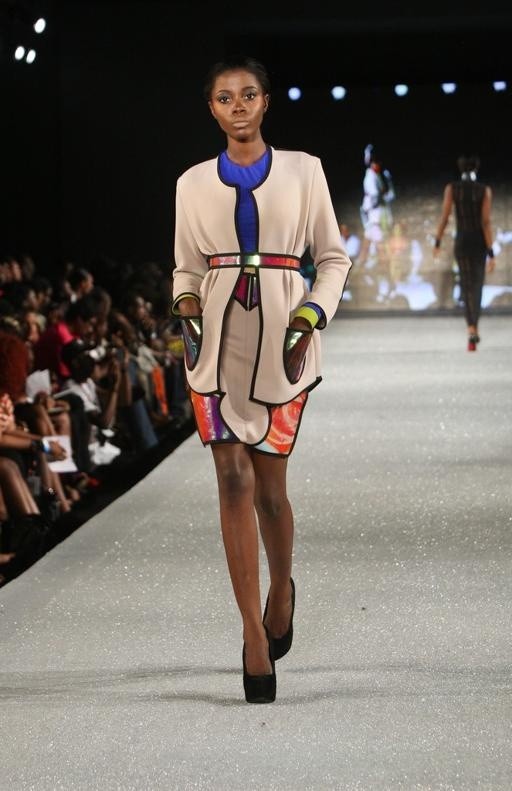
left=1, top=244, right=188, bottom=572
left=431, top=149, right=495, bottom=353
left=329, top=142, right=511, bottom=310
left=169, top=57, right=356, bottom=706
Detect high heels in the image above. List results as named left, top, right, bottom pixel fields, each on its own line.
left=468, top=334, right=480, bottom=351
left=243, top=576, right=296, bottom=703
